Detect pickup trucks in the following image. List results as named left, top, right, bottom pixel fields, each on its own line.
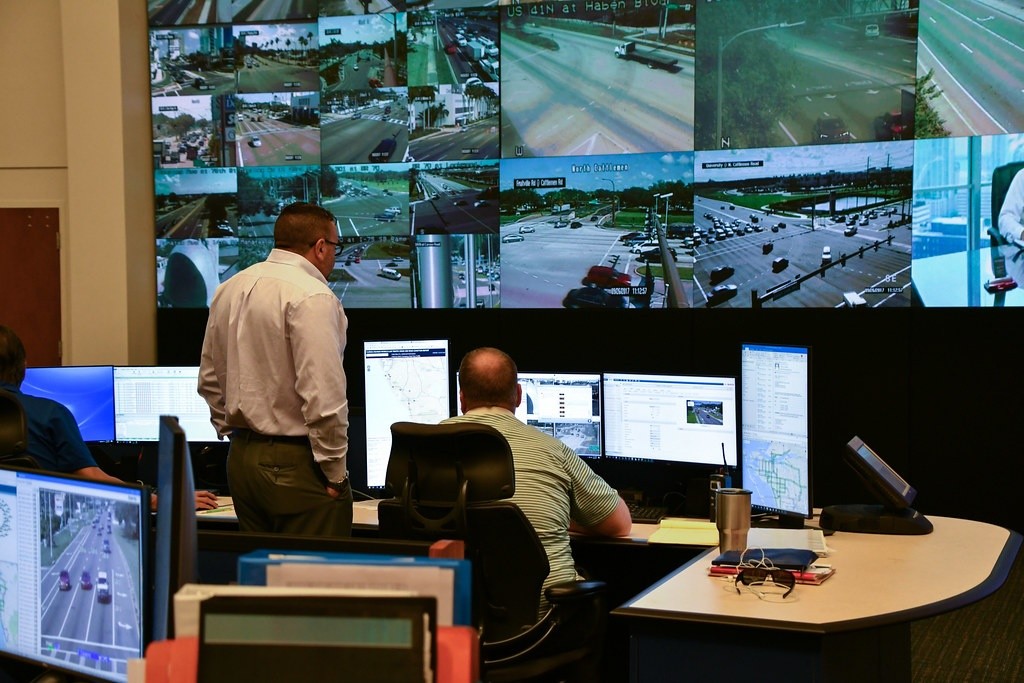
left=481, top=58, right=499, bottom=82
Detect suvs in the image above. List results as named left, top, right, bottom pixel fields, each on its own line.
left=195, top=78, right=206, bottom=88
left=96, top=572, right=110, bottom=602
left=252, top=135, right=261, bottom=146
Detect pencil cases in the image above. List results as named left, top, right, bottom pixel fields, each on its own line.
left=711, top=548, right=818, bottom=576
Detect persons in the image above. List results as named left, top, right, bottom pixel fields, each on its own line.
left=196, top=201, right=354, bottom=537
left=437, top=347, right=632, bottom=683
left=0, top=326, right=220, bottom=511
left=998, top=167, right=1024, bottom=243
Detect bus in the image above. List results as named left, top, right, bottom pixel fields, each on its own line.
left=478, top=36, right=499, bottom=57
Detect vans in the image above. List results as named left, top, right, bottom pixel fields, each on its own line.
left=238, top=114, right=243, bottom=121
left=772, top=256, right=790, bottom=271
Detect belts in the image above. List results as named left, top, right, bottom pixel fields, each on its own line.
left=232, top=431, right=308, bottom=446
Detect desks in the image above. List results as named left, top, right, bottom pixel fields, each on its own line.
left=143, top=487, right=1020, bottom=683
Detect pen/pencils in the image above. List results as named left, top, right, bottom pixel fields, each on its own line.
left=201, top=508, right=232, bottom=514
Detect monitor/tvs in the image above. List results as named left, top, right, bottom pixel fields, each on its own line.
left=361, top=337, right=814, bottom=530
left=843, top=435, right=917, bottom=510
left=0, top=365, right=231, bottom=683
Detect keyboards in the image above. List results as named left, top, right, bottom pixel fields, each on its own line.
left=626, top=505, right=668, bottom=524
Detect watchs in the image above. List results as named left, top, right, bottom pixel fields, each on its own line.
left=328, top=476, right=350, bottom=496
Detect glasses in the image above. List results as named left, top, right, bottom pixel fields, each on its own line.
left=310, top=238, right=345, bottom=257
left=735, top=569, right=795, bottom=599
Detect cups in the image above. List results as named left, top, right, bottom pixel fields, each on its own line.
left=714, top=487, right=753, bottom=556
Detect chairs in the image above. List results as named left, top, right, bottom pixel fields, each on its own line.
left=377, top=425, right=613, bottom=683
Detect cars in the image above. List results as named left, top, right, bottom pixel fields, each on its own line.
left=383, top=96, right=407, bottom=120
left=92, top=502, right=112, bottom=536
left=822, top=246, right=833, bottom=261
left=247, top=108, right=277, bottom=119
left=556, top=215, right=598, bottom=230
left=369, top=78, right=383, bottom=88
left=459, top=264, right=501, bottom=308
left=569, top=231, right=693, bottom=309
left=502, top=226, right=534, bottom=243
left=694, top=197, right=788, bottom=252
left=351, top=113, right=362, bottom=120
left=80, top=571, right=90, bottom=589
left=369, top=140, right=396, bottom=163
left=443, top=20, right=480, bottom=54
left=164, top=128, right=212, bottom=164
left=59, top=570, right=71, bottom=591
left=102, top=538, right=110, bottom=553
left=708, top=264, right=739, bottom=303
left=245, top=58, right=259, bottom=69
left=353, top=65, right=358, bottom=71
left=834, top=204, right=896, bottom=238
